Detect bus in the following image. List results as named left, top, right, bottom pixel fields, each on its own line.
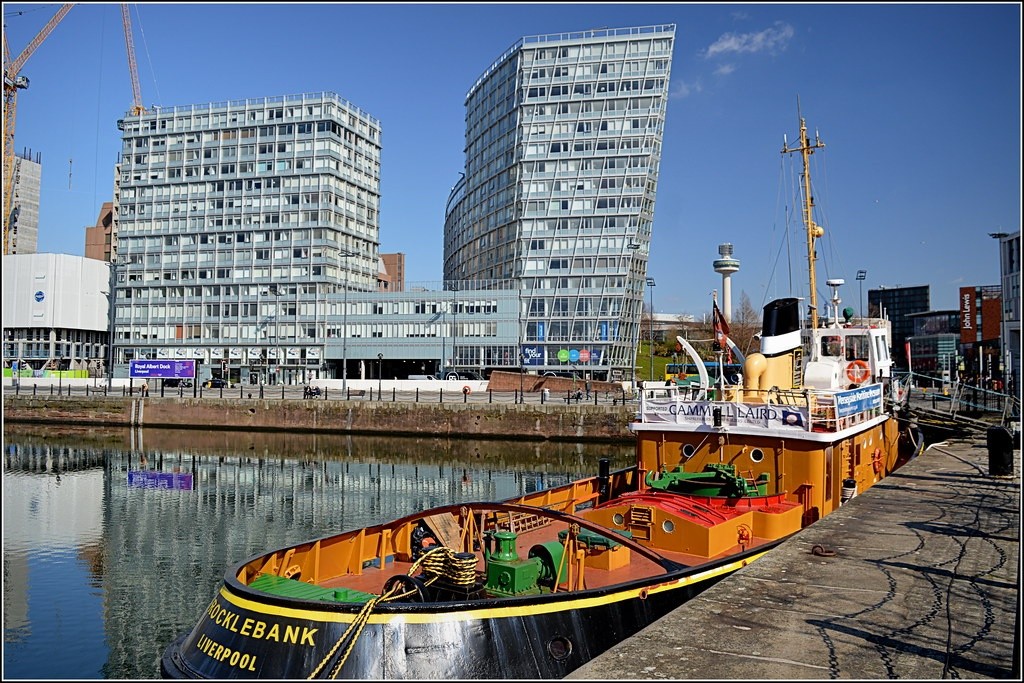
left=664, top=361, right=743, bottom=400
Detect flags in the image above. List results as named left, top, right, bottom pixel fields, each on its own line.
left=713, top=301, right=730, bottom=351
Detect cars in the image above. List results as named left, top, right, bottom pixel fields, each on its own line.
left=163, top=375, right=193, bottom=388
left=542, top=372, right=581, bottom=380
left=202, top=379, right=236, bottom=389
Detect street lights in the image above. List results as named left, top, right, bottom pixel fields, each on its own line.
left=645, top=275, right=657, bottom=381
left=854, top=268, right=868, bottom=320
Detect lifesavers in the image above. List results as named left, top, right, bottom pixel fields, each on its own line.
left=827, top=336, right=841, bottom=357
left=462, top=385, right=471, bottom=395
left=847, top=358, right=869, bottom=383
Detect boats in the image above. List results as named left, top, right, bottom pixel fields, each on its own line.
left=155, top=107, right=896, bottom=683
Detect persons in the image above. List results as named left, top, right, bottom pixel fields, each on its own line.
left=177, top=379, right=185, bottom=397
left=206, top=379, right=212, bottom=393
left=983, top=375, right=1014, bottom=393
left=140, top=382, right=150, bottom=397
left=251, top=372, right=256, bottom=386
left=664, top=377, right=673, bottom=398
left=303, top=386, right=323, bottom=399
left=922, top=385, right=927, bottom=401
left=569, top=388, right=583, bottom=399
left=585, top=380, right=592, bottom=400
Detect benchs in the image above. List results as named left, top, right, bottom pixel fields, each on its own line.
left=306, top=389, right=324, bottom=399
left=91, top=386, right=107, bottom=395
left=563, top=397, right=582, bottom=403
left=126, top=387, right=141, bottom=396
left=613, top=393, right=633, bottom=406
left=347, top=389, right=365, bottom=400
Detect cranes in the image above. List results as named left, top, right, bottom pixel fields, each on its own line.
left=0, top=3, right=157, bottom=253
